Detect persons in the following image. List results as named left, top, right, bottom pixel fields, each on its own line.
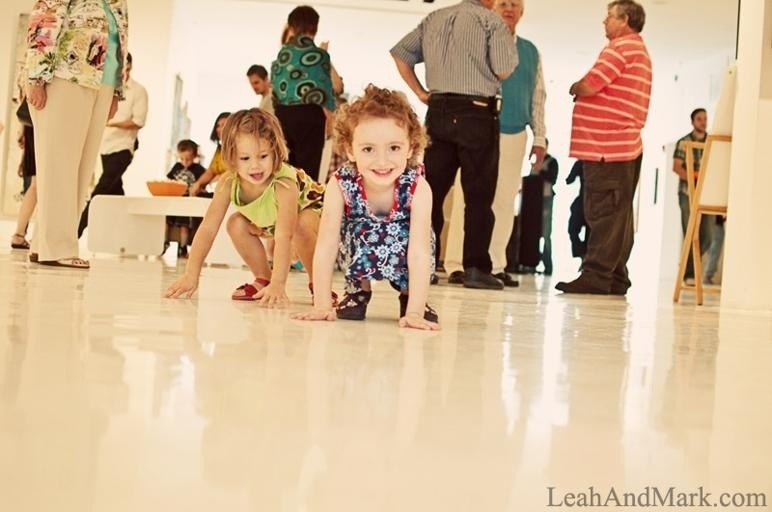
left=77, top=52, right=149, bottom=238
left=704, top=213, right=724, bottom=285
left=163, top=107, right=340, bottom=310
left=555, top=0, right=652, bottom=296
left=530, top=138, right=559, bottom=276
left=267, top=5, right=344, bottom=183
left=10, top=94, right=39, bottom=249
left=672, top=109, right=715, bottom=286
left=247, top=64, right=276, bottom=114
left=290, top=83, right=441, bottom=331
left=389, top=0, right=519, bottom=291
left=189, top=112, right=234, bottom=197
left=23, top=2, right=132, bottom=268
left=445, top=0, right=547, bottom=288
left=162, top=139, right=207, bottom=258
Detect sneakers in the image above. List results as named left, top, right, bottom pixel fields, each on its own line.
left=684, top=278, right=697, bottom=286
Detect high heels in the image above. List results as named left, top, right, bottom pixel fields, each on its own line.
left=331, top=285, right=376, bottom=325
left=397, top=290, right=440, bottom=331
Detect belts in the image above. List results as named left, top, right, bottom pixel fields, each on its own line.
left=428, top=89, right=497, bottom=109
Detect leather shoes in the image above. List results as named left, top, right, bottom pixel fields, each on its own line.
left=609, top=269, right=628, bottom=296
left=490, top=270, right=520, bottom=288
left=551, top=272, right=609, bottom=302
left=449, top=270, right=464, bottom=284
left=461, top=264, right=504, bottom=293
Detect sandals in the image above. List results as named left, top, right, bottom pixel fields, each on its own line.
left=228, top=275, right=275, bottom=303
left=11, top=234, right=30, bottom=249
left=39, top=255, right=90, bottom=274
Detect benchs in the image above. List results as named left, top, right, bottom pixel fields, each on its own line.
left=86, top=194, right=244, bottom=268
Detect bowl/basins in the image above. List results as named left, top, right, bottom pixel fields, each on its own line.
left=146, top=180, right=188, bottom=197
